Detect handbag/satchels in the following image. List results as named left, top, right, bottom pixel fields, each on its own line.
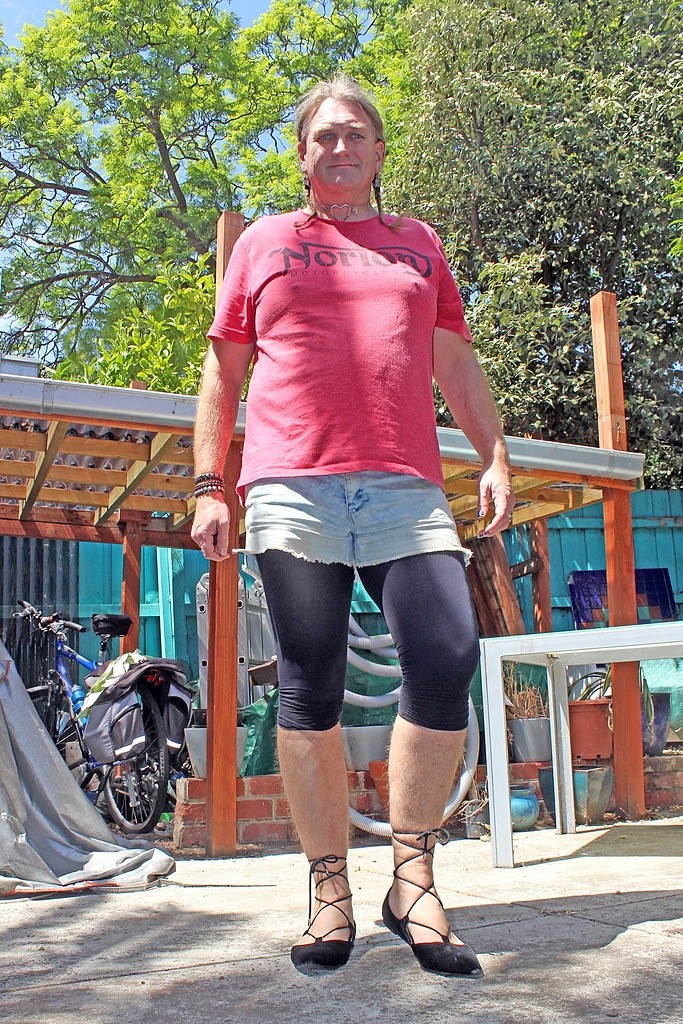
left=83, top=655, right=195, bottom=766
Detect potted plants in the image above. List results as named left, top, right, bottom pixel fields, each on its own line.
left=567, top=664, right=672, bottom=757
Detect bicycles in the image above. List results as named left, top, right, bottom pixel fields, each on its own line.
left=10, top=593, right=170, bottom=834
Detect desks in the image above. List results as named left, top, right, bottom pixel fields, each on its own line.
left=478, top=621, right=683, bottom=868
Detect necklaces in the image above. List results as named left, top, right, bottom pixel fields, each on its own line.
left=307, top=201, right=372, bottom=223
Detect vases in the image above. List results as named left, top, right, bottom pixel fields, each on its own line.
left=506, top=717, right=552, bottom=762
left=340, top=723, right=394, bottom=772
left=368, top=760, right=391, bottom=822
left=509, top=784, right=540, bottom=832
left=183, top=724, right=249, bottom=780
left=568, top=700, right=613, bottom=759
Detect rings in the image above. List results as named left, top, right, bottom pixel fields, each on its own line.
left=505, top=512, right=513, bottom=523
left=200, top=541, right=204, bottom=546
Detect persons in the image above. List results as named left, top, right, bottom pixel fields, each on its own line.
left=191, top=78, right=516, bottom=978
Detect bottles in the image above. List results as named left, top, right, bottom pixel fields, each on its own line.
left=71, top=683, right=90, bottom=726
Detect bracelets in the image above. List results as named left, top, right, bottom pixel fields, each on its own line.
left=194, top=473, right=224, bottom=498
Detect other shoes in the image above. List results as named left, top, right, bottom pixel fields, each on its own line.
left=382, top=829, right=483, bottom=979
left=291, top=856, right=356, bottom=976
